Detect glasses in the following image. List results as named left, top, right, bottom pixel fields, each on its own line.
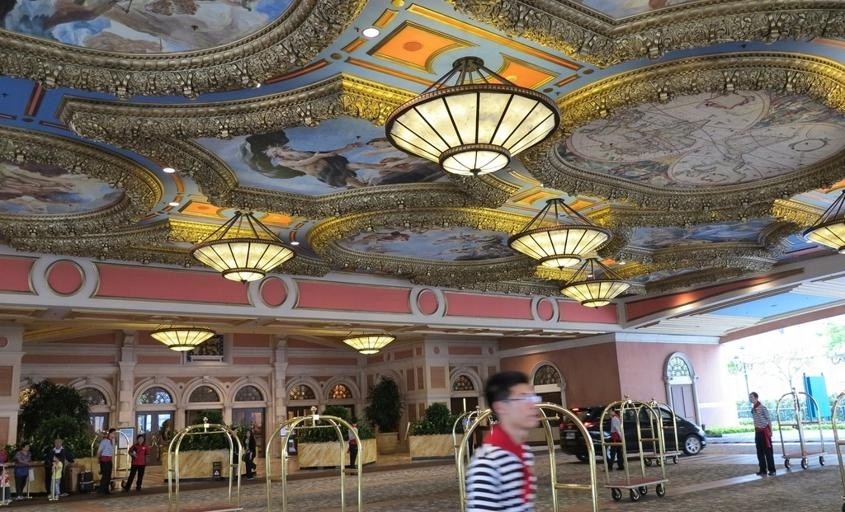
left=502, top=395, right=542, bottom=403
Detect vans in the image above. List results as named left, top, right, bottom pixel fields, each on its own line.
left=559, top=402, right=709, bottom=463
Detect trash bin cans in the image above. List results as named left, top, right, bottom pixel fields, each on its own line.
left=79, top=472, right=93, bottom=493
left=213, top=462, right=221, bottom=480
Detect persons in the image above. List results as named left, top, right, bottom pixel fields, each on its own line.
left=749, top=392, right=778, bottom=476
left=461, top=414, right=474, bottom=458
left=12, top=441, right=33, bottom=501
left=365, top=137, right=399, bottom=157
left=379, top=157, right=430, bottom=177
left=273, top=138, right=414, bottom=191
left=95, top=428, right=117, bottom=497
left=46, top=438, right=75, bottom=500
left=463, top=369, right=543, bottom=511
left=121, top=434, right=149, bottom=492
left=600, top=408, right=626, bottom=472
left=346, top=415, right=360, bottom=475
left=241, top=428, right=257, bottom=480
left=47, top=453, right=63, bottom=501
left=0, top=466, right=12, bottom=503
left=226, top=427, right=238, bottom=482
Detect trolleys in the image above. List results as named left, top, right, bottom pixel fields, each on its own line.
left=91, top=430, right=131, bottom=491
left=832, top=392, right=845, bottom=511
left=637, top=398, right=682, bottom=468
left=598, top=395, right=669, bottom=500
left=167, top=416, right=243, bottom=512
left=450, top=404, right=599, bottom=512
left=263, top=406, right=362, bottom=512
left=775, top=386, right=827, bottom=470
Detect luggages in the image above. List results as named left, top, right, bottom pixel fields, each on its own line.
left=66, top=463, right=94, bottom=492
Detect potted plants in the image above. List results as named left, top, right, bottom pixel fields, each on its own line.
left=362, top=375, right=406, bottom=455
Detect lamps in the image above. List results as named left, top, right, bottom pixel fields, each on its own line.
left=341, top=328, right=395, bottom=355
left=191, top=211, right=296, bottom=284
left=803, top=190, right=845, bottom=254
left=384, top=56, right=561, bottom=177
left=508, top=198, right=631, bottom=310
left=150, top=319, right=217, bottom=354
